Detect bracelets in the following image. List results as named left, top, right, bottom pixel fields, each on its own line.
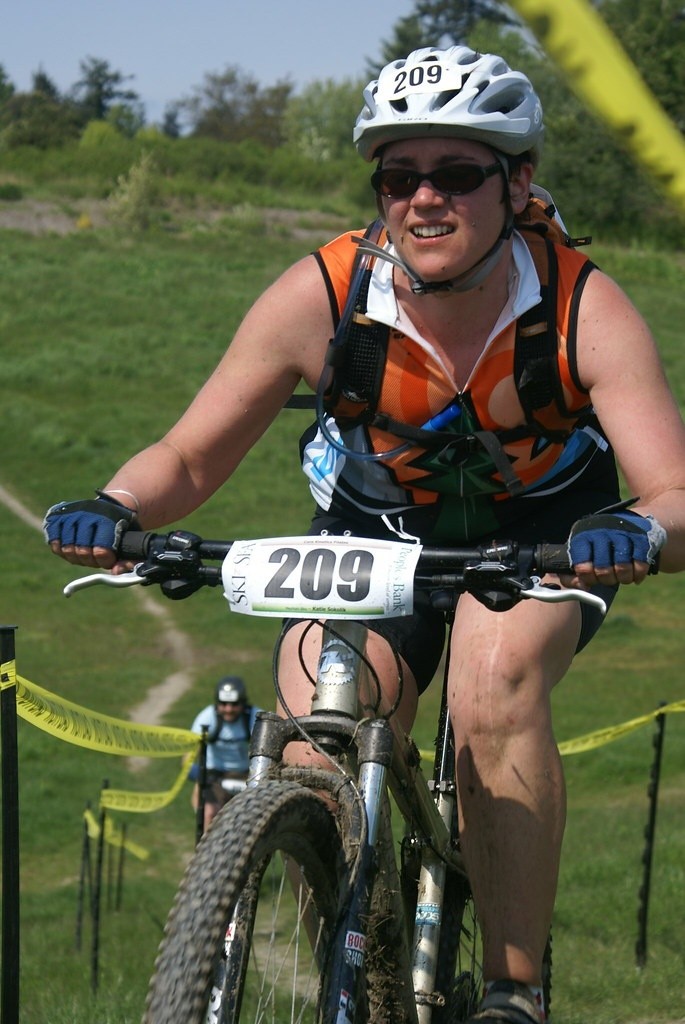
left=94, top=490, right=140, bottom=512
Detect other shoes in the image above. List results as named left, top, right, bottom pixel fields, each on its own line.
left=469, top=979, right=539, bottom=1024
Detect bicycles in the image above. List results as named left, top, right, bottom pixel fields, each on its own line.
left=60, top=528, right=666, bottom=1024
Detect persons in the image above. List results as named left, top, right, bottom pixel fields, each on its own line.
left=182, top=676, right=266, bottom=830
left=42, top=44, right=685, bottom=1024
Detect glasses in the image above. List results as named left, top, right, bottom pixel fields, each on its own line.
left=370, top=159, right=503, bottom=200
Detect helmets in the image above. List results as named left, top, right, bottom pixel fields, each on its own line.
left=352, top=47, right=544, bottom=154
left=214, top=678, right=244, bottom=704
